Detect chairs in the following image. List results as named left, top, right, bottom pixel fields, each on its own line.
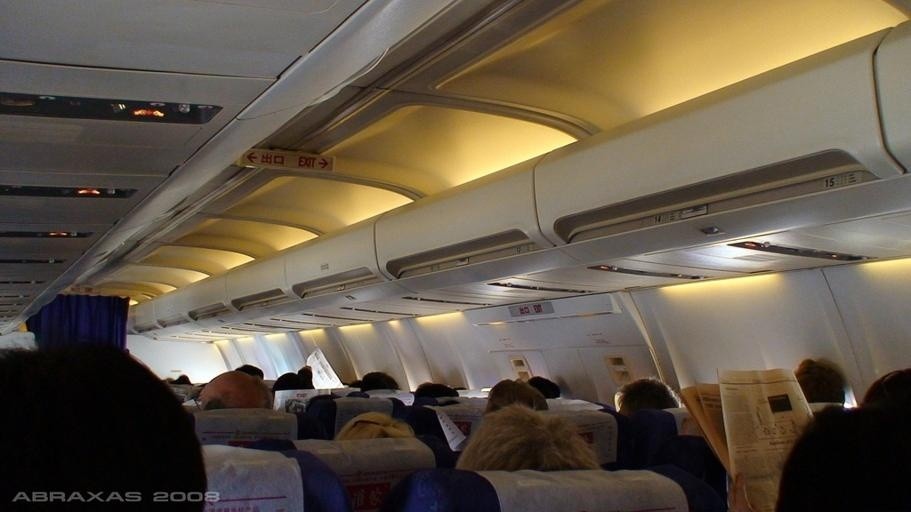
left=167, top=378, right=911, bottom=512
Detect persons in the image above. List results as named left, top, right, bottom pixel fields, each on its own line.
left=1, top=346, right=911, bottom=512
